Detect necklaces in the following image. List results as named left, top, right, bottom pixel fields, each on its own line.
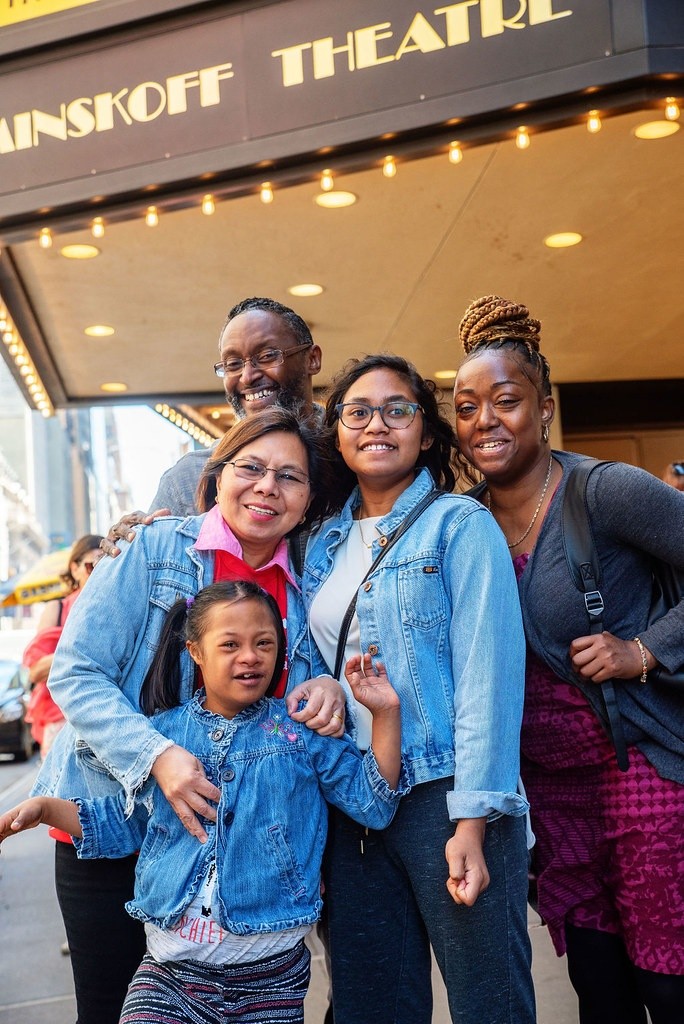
left=347, top=483, right=437, bottom=549
left=480, top=454, right=556, bottom=548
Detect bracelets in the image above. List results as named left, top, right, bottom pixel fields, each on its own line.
left=631, top=636, right=651, bottom=684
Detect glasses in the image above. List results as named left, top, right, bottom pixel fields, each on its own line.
left=335, top=401, right=421, bottom=430
left=214, top=344, right=312, bottom=377
left=221, top=460, right=314, bottom=491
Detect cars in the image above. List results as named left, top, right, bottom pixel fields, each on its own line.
left=0, top=647, right=44, bottom=762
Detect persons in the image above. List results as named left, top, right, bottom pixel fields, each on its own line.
left=0, top=582, right=404, bottom=1024
left=38, top=407, right=341, bottom=1024
left=98, top=294, right=339, bottom=561
left=438, top=295, right=683, bottom=1024
left=288, top=350, right=561, bottom=1023
left=16, top=532, right=113, bottom=948
